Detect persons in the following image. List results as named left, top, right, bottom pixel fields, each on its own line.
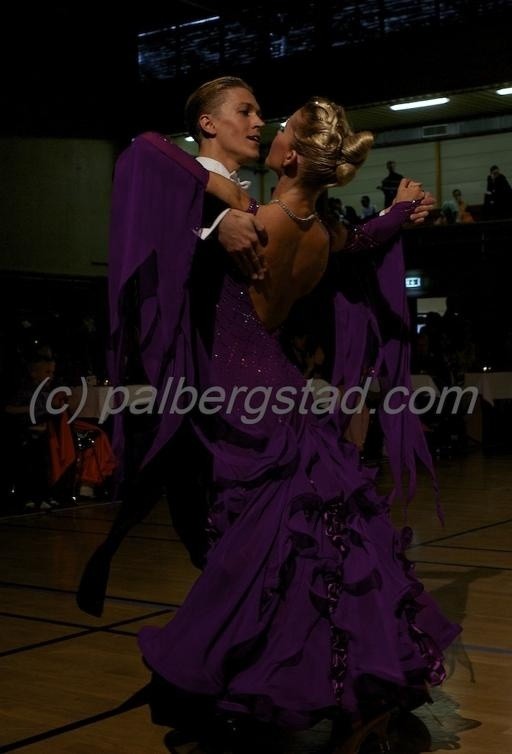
left=0, top=157, right=512, bottom=516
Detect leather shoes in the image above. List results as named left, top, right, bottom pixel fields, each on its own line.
left=76, top=553, right=110, bottom=617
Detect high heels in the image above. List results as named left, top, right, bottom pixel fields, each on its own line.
left=326, top=698, right=394, bottom=752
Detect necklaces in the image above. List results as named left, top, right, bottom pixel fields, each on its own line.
left=266, top=198, right=323, bottom=227
